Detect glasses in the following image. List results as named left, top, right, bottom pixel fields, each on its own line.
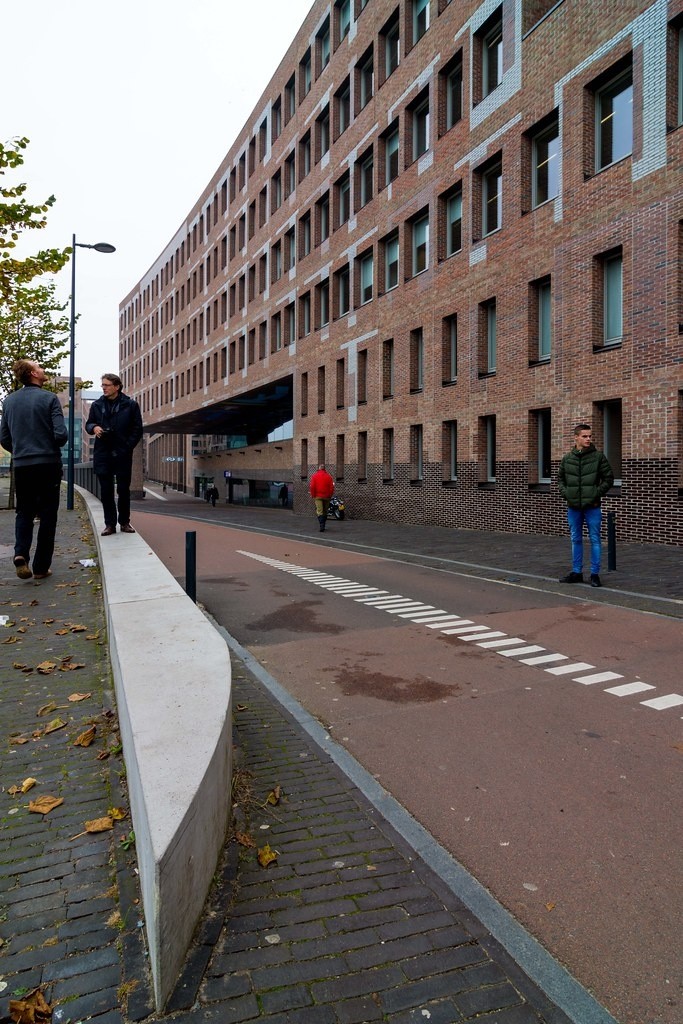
left=101, top=384, right=113, bottom=388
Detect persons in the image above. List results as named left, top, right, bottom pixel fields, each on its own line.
left=0, top=359, right=69, bottom=581
left=279, top=483, right=288, bottom=509
left=210, top=484, right=219, bottom=507
left=557, top=424, right=615, bottom=586
left=84, top=373, right=141, bottom=537
left=310, top=464, right=335, bottom=533
left=162, top=481, right=167, bottom=494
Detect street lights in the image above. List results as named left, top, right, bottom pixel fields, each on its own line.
left=65, top=232, right=117, bottom=511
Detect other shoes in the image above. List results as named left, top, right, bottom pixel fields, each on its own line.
left=34, top=569, right=52, bottom=580
left=13, top=555, right=32, bottom=580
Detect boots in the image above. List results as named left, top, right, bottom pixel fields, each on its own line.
left=318, top=514, right=327, bottom=532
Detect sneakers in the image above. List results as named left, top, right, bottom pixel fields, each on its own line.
left=120, top=524, right=135, bottom=533
left=590, top=574, right=601, bottom=588
left=559, top=572, right=584, bottom=583
left=101, top=525, right=116, bottom=536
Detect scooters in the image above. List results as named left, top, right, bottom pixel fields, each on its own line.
left=307, top=482, right=346, bottom=522
